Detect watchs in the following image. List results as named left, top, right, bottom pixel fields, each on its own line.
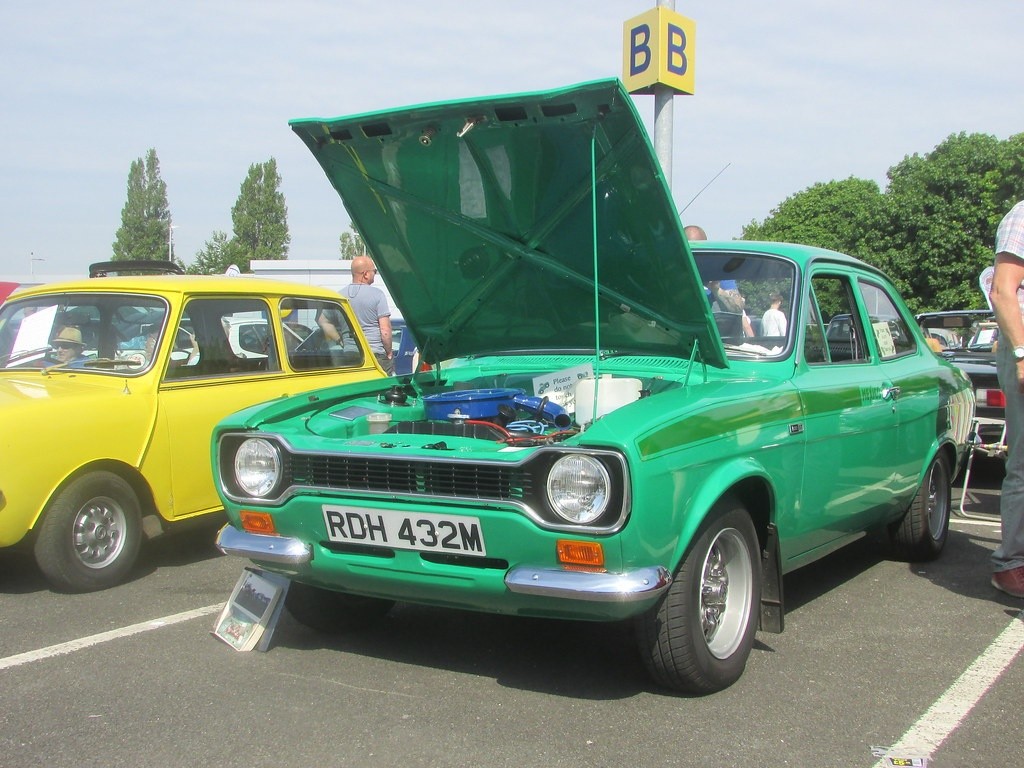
left=1012, top=346, right=1024, bottom=360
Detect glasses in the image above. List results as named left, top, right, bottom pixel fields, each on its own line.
left=53, top=342, right=77, bottom=349
left=364, top=269, right=377, bottom=274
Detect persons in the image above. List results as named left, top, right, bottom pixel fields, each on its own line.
left=757, top=294, right=787, bottom=336
left=314, top=256, right=396, bottom=376
left=989, top=197, right=1024, bottom=596
left=683, top=225, right=741, bottom=313
left=34, top=326, right=94, bottom=369
left=740, top=297, right=755, bottom=338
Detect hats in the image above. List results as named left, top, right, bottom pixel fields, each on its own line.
left=52, top=327, right=86, bottom=346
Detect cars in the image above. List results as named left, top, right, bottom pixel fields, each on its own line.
left=0, top=260, right=388, bottom=590
left=916, top=309, right=1013, bottom=489
left=210, top=78, right=976, bottom=699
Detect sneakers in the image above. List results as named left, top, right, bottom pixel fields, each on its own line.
left=991, top=566, right=1024, bottom=598
